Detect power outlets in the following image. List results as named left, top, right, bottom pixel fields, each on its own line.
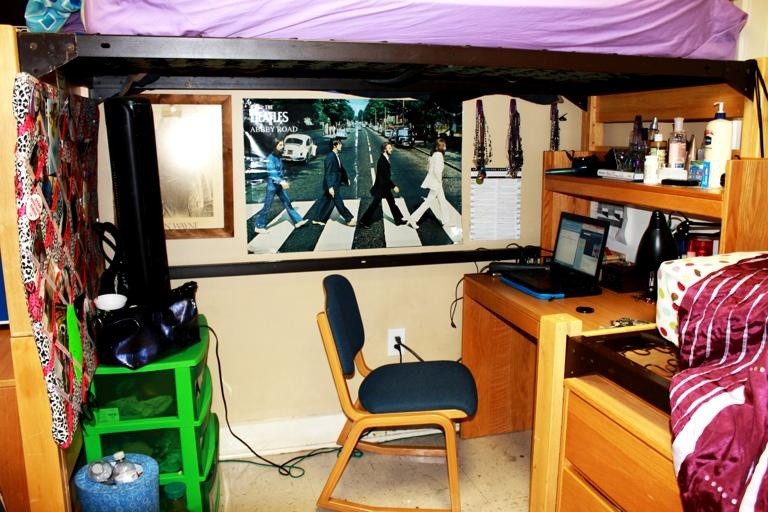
left=387, top=327, right=407, bottom=357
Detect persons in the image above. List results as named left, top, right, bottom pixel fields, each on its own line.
left=407, top=138, right=458, bottom=230
left=360, top=143, right=407, bottom=229
left=311, top=137, right=358, bottom=226
left=254, top=140, right=309, bottom=234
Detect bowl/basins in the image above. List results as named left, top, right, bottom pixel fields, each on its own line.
left=573, top=157, right=606, bottom=177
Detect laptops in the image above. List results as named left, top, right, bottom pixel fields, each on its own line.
left=500, top=211, right=610, bottom=300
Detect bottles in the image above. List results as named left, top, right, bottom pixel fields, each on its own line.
left=88, top=450, right=139, bottom=486
left=629, top=113, right=686, bottom=170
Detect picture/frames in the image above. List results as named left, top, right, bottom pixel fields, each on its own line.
left=130, top=94, right=235, bottom=241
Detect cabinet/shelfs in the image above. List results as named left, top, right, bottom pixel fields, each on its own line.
left=79, top=313, right=221, bottom=511
left=458, top=81, right=768, bottom=441
left=529, top=158, right=768, bottom=512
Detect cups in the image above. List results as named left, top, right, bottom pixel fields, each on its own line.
left=93, top=292, right=128, bottom=325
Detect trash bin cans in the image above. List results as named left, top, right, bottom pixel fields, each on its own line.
left=74, top=453, right=159, bottom=512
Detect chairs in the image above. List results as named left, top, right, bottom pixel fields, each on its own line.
left=316, top=275, right=478, bottom=512
